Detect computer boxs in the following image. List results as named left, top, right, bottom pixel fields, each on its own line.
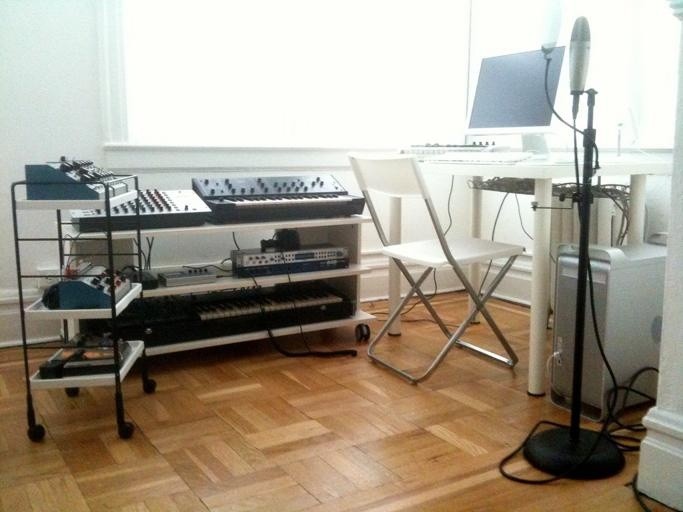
left=550, top=232, right=669, bottom=423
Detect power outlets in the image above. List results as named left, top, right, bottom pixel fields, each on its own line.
left=34, top=266, right=60, bottom=290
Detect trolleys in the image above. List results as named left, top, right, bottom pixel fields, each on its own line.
left=11, top=155, right=157, bottom=443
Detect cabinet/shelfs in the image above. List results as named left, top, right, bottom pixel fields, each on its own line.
left=51, top=216, right=377, bottom=397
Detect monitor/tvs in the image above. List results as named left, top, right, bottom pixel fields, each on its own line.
left=464, top=45, right=568, bottom=135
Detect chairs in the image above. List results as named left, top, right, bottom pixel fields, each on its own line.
left=346, top=153, right=527, bottom=385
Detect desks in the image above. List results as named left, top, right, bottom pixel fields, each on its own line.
left=387, top=158, right=673, bottom=397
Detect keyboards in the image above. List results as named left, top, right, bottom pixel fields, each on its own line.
left=422, top=152, right=531, bottom=165
